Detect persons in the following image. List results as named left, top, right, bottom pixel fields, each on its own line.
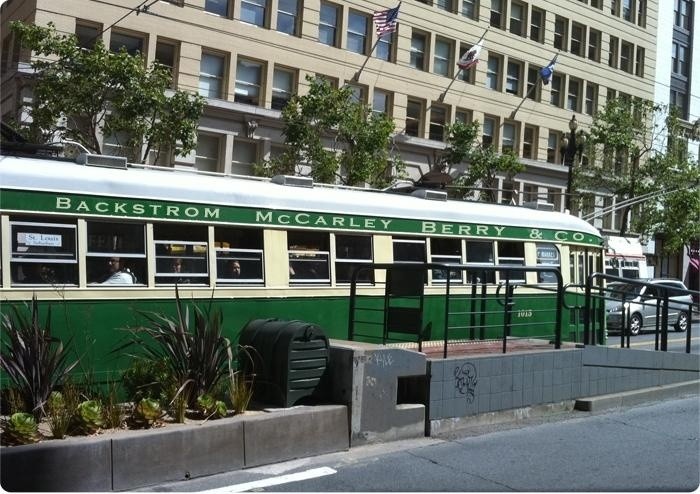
left=83, top=256, right=297, bottom=285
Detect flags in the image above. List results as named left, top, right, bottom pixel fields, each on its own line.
left=373, top=8, right=399, bottom=38
left=456, top=38, right=484, bottom=70
left=540, top=54, right=559, bottom=86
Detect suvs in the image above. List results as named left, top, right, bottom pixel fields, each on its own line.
left=604, top=277, right=694, bottom=335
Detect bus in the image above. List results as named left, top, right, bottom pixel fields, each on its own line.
left=0, top=120, right=604, bottom=415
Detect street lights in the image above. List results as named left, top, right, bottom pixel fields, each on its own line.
left=557, top=110, right=586, bottom=213
